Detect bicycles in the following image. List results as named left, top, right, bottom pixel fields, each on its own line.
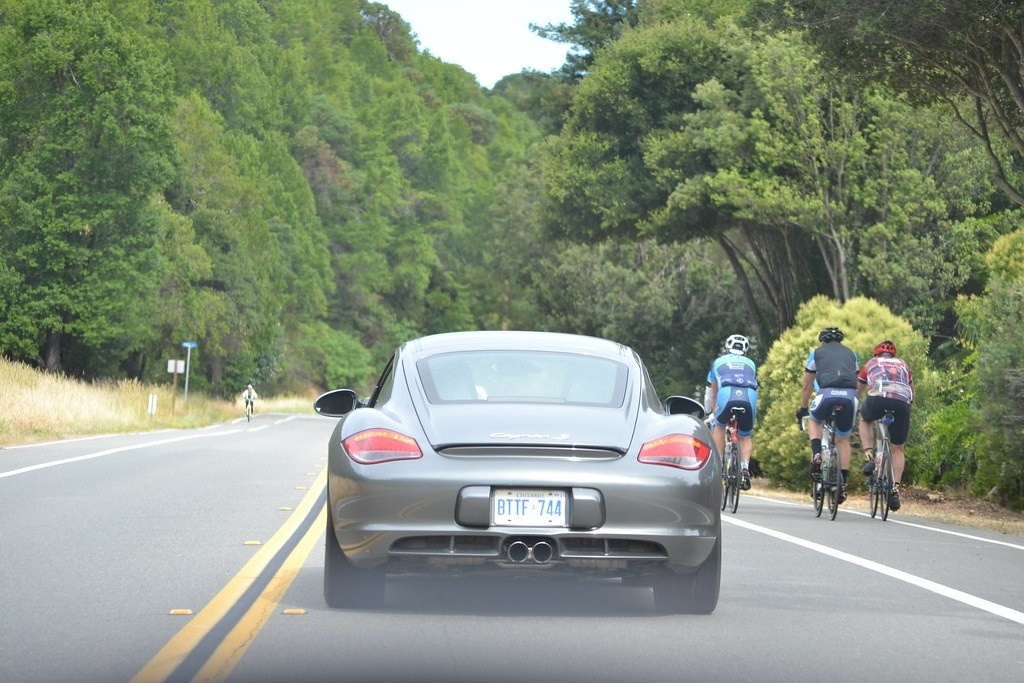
left=706, top=405, right=750, bottom=514
left=855, top=409, right=899, bottom=522
left=798, top=405, right=847, bottom=521
left=245, top=396, right=257, bottom=421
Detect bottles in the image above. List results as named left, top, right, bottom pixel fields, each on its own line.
left=725, top=442, right=731, bottom=464
left=876, top=452, right=883, bottom=474
left=820, top=449, right=830, bottom=481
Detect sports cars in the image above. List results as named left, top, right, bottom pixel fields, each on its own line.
left=315, top=329, right=726, bottom=616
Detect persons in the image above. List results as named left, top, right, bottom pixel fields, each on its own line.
left=796, top=327, right=860, bottom=504
left=856, top=340, right=914, bottom=512
left=242, top=384, right=257, bottom=420
left=704, top=334, right=758, bottom=492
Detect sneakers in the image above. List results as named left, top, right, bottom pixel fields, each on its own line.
left=889, top=491, right=900, bottom=511
left=811, top=453, right=822, bottom=482
left=838, top=492, right=848, bottom=504
left=742, top=469, right=751, bottom=490
left=862, top=459, right=875, bottom=477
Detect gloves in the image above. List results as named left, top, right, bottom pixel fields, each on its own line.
left=796, top=407, right=808, bottom=424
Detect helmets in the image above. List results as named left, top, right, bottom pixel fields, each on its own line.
left=724, top=334, right=751, bottom=355
left=873, top=341, right=897, bottom=357
left=819, top=327, right=844, bottom=342
